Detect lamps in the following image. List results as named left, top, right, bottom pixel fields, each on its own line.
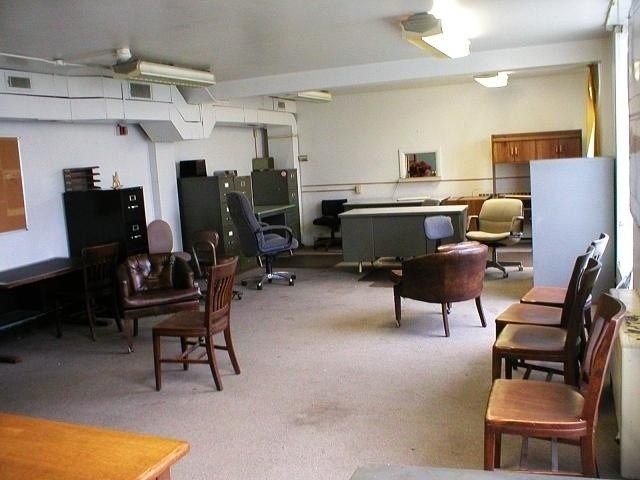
left=111, top=56, right=216, bottom=88
left=398, top=12, right=470, bottom=59
left=473, top=71, right=508, bottom=87
left=294, top=90, right=333, bottom=104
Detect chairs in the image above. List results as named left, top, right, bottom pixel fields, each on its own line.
left=313, top=199, right=347, bottom=251
left=190, top=231, right=241, bottom=300
left=423, top=215, right=455, bottom=254
left=421, top=199, right=440, bottom=206
left=390, top=243, right=489, bottom=337
left=226, top=192, right=299, bottom=290
left=55, top=241, right=124, bottom=342
left=483, top=232, right=628, bottom=478
left=147, top=219, right=192, bottom=262
left=116, top=252, right=203, bottom=355
left=465, top=198, right=525, bottom=278
left=152, top=255, right=241, bottom=391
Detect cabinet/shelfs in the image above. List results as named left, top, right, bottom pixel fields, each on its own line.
left=490, top=129, right=582, bottom=163
left=62, top=186, right=148, bottom=319
left=251, top=169, right=301, bottom=245
left=177, top=175, right=254, bottom=275
left=338, top=205, right=468, bottom=274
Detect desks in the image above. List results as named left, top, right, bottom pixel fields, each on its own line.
left=0, top=257, right=80, bottom=338
left=0, top=411, right=191, bottom=479
left=253, top=204, right=297, bottom=239
left=349, top=464, right=555, bottom=480
